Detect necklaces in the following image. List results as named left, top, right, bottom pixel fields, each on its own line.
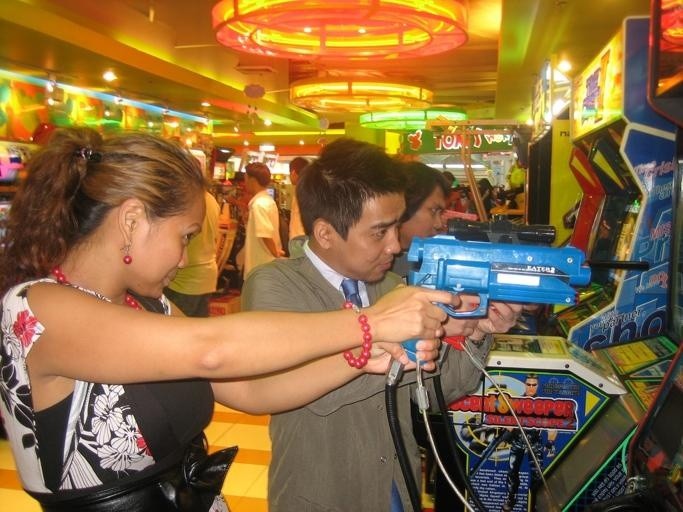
left=51, top=265, right=144, bottom=313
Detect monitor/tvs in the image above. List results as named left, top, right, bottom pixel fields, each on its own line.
left=588, top=191, right=627, bottom=281
left=606, top=195, right=643, bottom=287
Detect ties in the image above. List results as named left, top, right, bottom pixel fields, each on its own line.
left=338, top=276, right=364, bottom=310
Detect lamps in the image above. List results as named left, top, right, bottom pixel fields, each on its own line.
left=209, top=0, right=469, bottom=131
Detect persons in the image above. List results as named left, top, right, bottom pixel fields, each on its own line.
left=455, top=185, right=509, bottom=220
left=163, top=190, right=220, bottom=318
left=477, top=178, right=495, bottom=218
left=289, top=157, right=310, bottom=241
left=442, top=170, right=466, bottom=212
left=243, top=162, right=285, bottom=279
left=229, top=172, right=254, bottom=233
left=0, top=124, right=463, bottom=511
left=237, top=134, right=540, bottom=510
left=386, top=156, right=448, bottom=284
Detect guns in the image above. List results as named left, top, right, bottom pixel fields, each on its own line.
left=466, top=428, right=511, bottom=481
left=403, top=220, right=648, bottom=364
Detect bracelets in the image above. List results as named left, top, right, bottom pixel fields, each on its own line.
left=338, top=300, right=373, bottom=370
